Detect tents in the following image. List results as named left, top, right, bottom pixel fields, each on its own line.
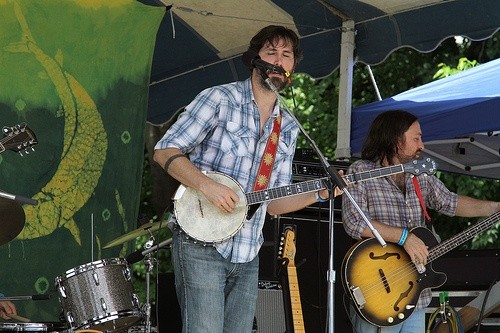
left=347, top=57, right=500, bottom=182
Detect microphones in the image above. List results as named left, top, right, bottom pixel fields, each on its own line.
left=126, top=238, right=173, bottom=264
left=242, top=51, right=287, bottom=76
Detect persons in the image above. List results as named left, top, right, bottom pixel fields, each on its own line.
left=342, top=109, right=500, bottom=333
left=0, top=293, right=17, bottom=321
left=153, top=25, right=350, bottom=333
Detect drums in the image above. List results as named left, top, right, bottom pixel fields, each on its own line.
left=0, top=320, right=62, bottom=333
left=53, top=256, right=147, bottom=332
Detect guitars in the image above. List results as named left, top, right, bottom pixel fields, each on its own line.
left=172, top=156, right=438, bottom=247
left=277, top=223, right=306, bottom=333
left=340, top=209, right=500, bottom=327
left=0, top=121, right=39, bottom=157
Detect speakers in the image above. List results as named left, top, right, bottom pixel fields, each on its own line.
left=250, top=215, right=358, bottom=333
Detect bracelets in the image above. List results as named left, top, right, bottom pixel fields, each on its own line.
left=314, top=191, right=329, bottom=203
left=398, top=227, right=408, bottom=246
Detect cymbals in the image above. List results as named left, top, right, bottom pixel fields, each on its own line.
left=100, top=217, right=168, bottom=250
left=0, top=197, right=26, bottom=245
